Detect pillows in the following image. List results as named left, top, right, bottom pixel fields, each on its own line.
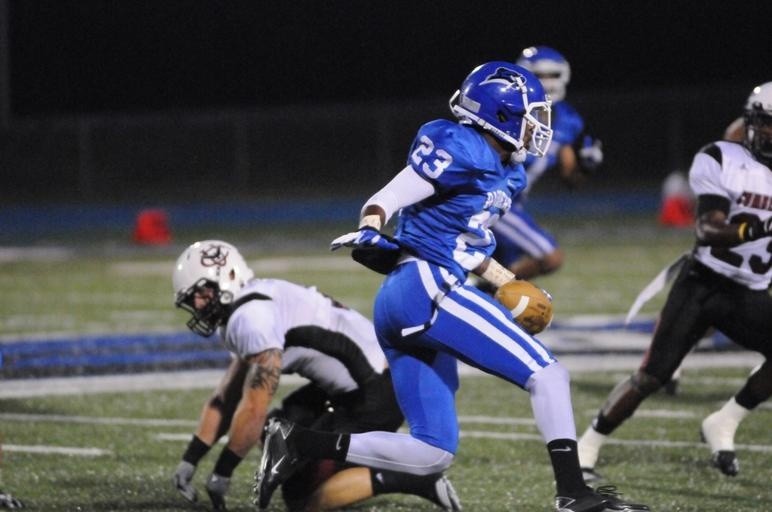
left=515, top=47, right=572, bottom=105
left=447, top=59, right=553, bottom=158
left=173, top=239, right=253, bottom=338
left=744, top=81, right=772, bottom=165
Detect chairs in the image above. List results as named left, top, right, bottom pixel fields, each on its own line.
left=417, top=470, right=461, bottom=512
left=698, top=411, right=741, bottom=476
left=252, top=417, right=303, bottom=510
left=579, top=467, right=610, bottom=488
left=555, top=485, right=649, bottom=512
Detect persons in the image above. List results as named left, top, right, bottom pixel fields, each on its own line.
left=254, top=61, right=651, bottom=512
left=640, top=115, right=745, bottom=396
left=463, top=45, right=604, bottom=299
left=577, top=81, right=772, bottom=491
left=170, top=239, right=462, bottom=512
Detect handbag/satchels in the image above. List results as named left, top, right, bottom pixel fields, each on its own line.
left=357, top=213, right=383, bottom=233
left=212, top=447, right=243, bottom=479
left=480, top=257, right=516, bottom=290
left=181, top=434, right=211, bottom=467
left=737, top=217, right=748, bottom=245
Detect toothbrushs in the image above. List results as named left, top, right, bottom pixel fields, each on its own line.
left=494, top=279, right=553, bottom=335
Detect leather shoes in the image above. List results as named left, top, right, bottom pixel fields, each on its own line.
left=172, top=462, right=200, bottom=505
left=330, top=223, right=400, bottom=252
left=577, top=140, right=604, bottom=178
left=205, top=471, right=232, bottom=510
